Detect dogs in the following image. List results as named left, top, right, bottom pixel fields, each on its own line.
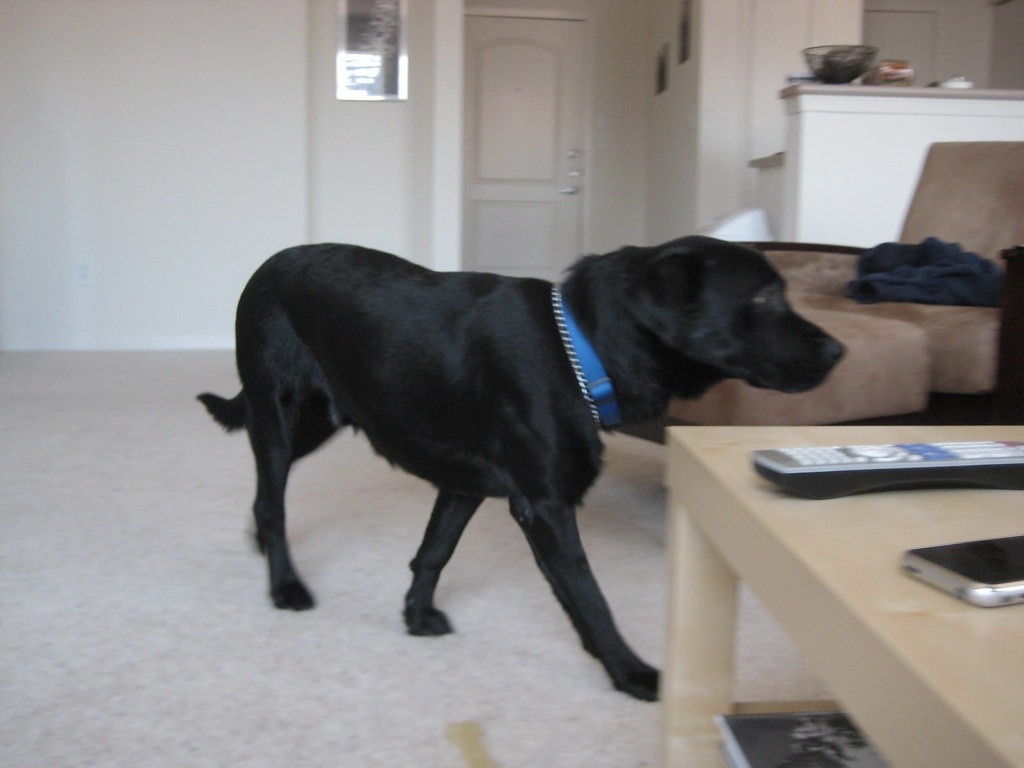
left=196, top=234, right=850, bottom=703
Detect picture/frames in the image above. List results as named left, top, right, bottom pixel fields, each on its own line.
left=335, top=0, right=409, bottom=101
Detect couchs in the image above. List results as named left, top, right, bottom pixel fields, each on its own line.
left=620, top=141, right=1024, bottom=442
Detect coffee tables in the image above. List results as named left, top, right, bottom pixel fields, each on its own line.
left=657, top=425, right=1024, bottom=768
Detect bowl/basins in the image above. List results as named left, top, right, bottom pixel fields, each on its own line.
left=801, top=45, right=879, bottom=84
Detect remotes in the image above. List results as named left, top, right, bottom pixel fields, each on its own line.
left=751, top=439, right=1024, bottom=500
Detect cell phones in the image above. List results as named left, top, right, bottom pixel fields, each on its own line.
left=903, top=536, right=1024, bottom=609
